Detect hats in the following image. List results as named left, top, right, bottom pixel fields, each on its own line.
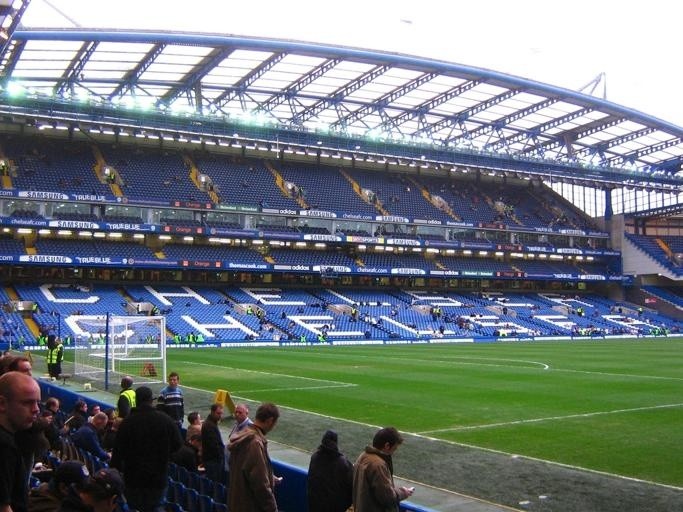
left=53, top=460, right=127, bottom=500
left=321, top=430, right=338, bottom=448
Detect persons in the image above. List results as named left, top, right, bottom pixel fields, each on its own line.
left=346, top=426, right=415, bottom=512
left=227, top=402, right=281, bottom=512
left=306, top=429, right=356, bottom=511
left=54, top=336, right=64, bottom=375
left=47, top=333, right=62, bottom=380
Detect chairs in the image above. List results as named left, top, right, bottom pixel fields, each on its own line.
left=26, top=400, right=228, bottom=511
left=0, top=130, right=683, bottom=344
